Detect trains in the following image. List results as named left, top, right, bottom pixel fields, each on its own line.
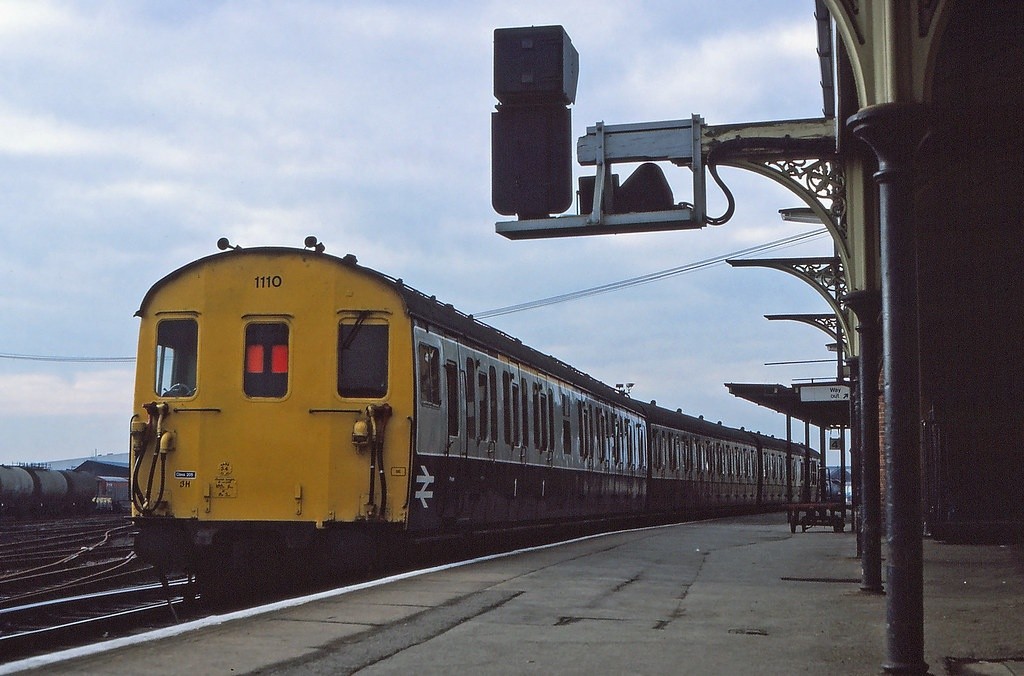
left=127, top=237, right=822, bottom=604
